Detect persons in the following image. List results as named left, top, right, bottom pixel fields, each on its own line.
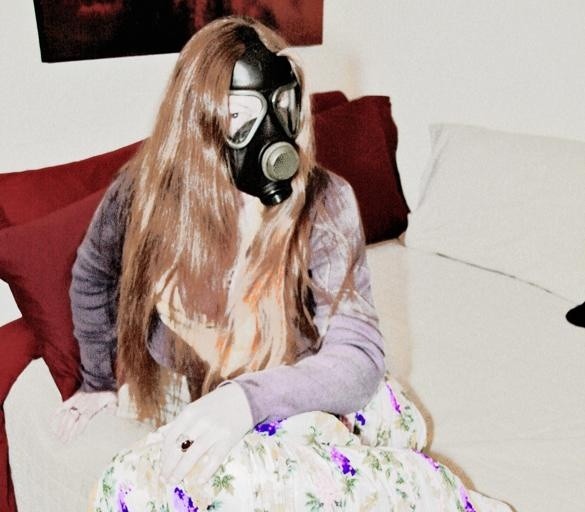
left=45, top=13, right=386, bottom=487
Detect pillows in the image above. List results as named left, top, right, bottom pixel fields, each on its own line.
left=308, top=90, right=349, bottom=115
left=1, top=183, right=115, bottom=402
left=1, top=136, right=148, bottom=232
left=308, top=98, right=411, bottom=252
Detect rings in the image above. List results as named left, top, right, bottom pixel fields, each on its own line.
left=181, top=438, right=194, bottom=454
left=70, top=406, right=77, bottom=414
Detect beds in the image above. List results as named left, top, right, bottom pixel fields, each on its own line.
left=2, top=238, right=585, bottom=510
